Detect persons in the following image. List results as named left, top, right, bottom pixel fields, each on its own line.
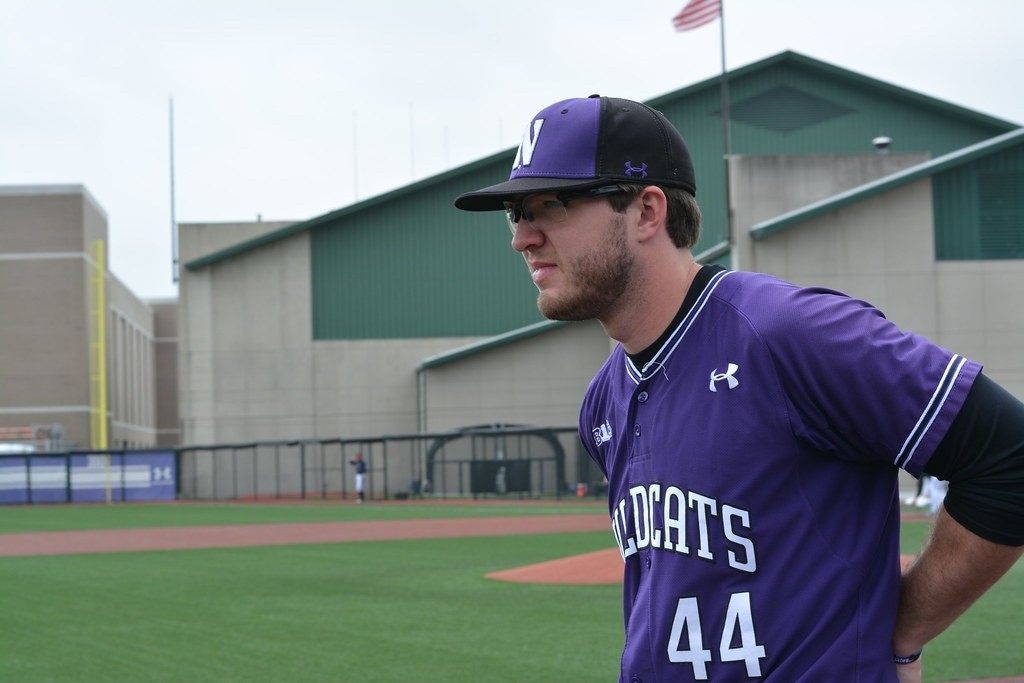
left=349, top=454, right=367, bottom=503
left=454, top=96, right=1024, bottom=682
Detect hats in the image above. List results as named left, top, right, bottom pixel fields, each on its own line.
left=455, top=95, right=697, bottom=213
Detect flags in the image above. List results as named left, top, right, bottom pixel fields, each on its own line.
left=673, top=0, right=721, bottom=33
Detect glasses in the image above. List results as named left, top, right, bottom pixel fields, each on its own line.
left=502, top=184, right=671, bottom=236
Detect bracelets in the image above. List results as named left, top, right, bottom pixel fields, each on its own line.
left=894, top=650, right=923, bottom=665
